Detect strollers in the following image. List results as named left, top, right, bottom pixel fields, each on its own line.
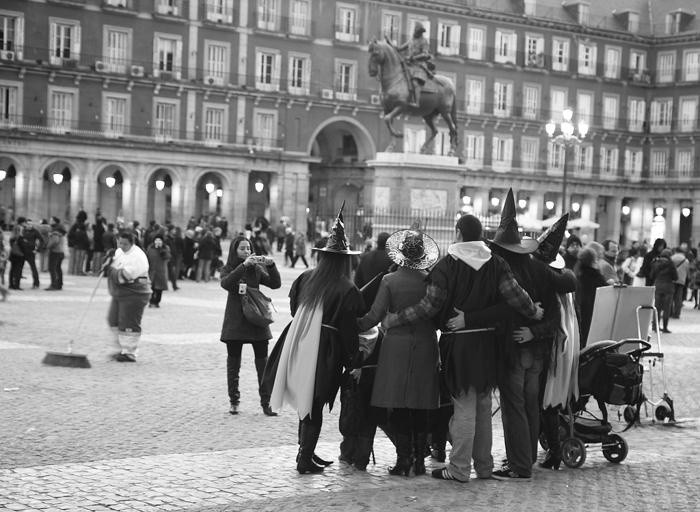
left=538, top=338, right=652, bottom=467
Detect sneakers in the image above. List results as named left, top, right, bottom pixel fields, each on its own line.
left=338, top=447, right=371, bottom=471
left=111, top=353, right=138, bottom=363
left=431, top=467, right=468, bottom=482
left=490, top=463, right=533, bottom=482
left=430, top=447, right=446, bottom=462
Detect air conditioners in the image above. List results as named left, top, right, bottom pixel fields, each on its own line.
left=205, top=77, right=215, bottom=86
left=371, top=96, right=380, bottom=104
left=1, top=51, right=14, bottom=62
left=96, top=62, right=110, bottom=72
left=322, top=89, right=333, bottom=99
left=131, top=66, right=143, bottom=77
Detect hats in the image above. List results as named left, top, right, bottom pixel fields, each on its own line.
left=531, top=212, right=570, bottom=271
left=384, top=218, right=442, bottom=271
left=309, top=199, right=364, bottom=255
left=486, top=188, right=539, bottom=255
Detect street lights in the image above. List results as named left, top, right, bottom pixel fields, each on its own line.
left=541, top=105, right=590, bottom=244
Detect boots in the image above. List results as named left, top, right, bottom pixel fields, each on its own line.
left=414, top=430, right=427, bottom=476
left=227, top=356, right=241, bottom=414
left=539, top=414, right=563, bottom=471
left=253, top=355, right=277, bottom=416
left=388, top=431, right=412, bottom=476
left=653, top=316, right=672, bottom=334
left=297, top=420, right=333, bottom=475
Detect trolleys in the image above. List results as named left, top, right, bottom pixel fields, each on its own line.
left=616, top=304, right=678, bottom=431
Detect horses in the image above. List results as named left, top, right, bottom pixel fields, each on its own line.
left=367, top=36, right=459, bottom=156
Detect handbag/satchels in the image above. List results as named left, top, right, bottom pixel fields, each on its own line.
left=241, top=286, right=276, bottom=328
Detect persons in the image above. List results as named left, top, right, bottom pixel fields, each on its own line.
left=380, top=20, right=439, bottom=111
left=8, top=204, right=111, bottom=291
left=244, top=211, right=308, bottom=268
left=572, top=238, right=700, bottom=350
left=287, top=215, right=580, bottom=482
left=104, top=213, right=227, bottom=307
left=219, top=237, right=278, bottom=416
left=106, top=232, right=151, bottom=362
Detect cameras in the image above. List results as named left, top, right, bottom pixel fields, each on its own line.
left=253, top=255, right=265, bottom=264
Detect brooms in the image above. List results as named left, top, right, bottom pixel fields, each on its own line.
left=40, top=271, right=104, bottom=368
